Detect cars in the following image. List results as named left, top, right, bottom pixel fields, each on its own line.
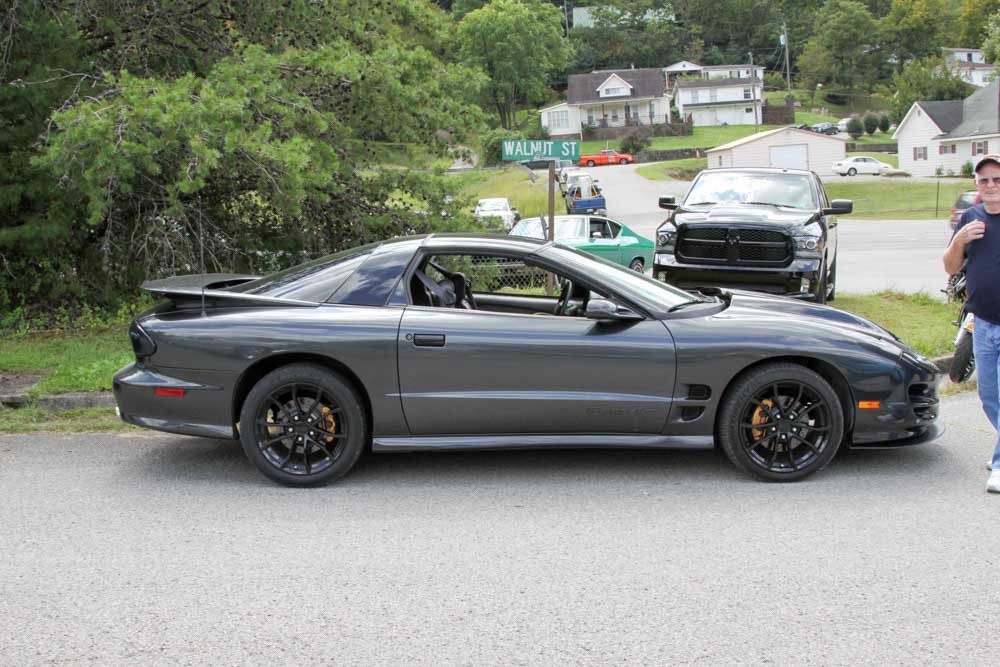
left=832, top=156, right=894, bottom=176
left=110, top=232, right=938, bottom=484
left=555, top=159, right=606, bottom=215
left=824, top=93, right=847, bottom=104
left=509, top=215, right=655, bottom=273
left=470, top=198, right=516, bottom=232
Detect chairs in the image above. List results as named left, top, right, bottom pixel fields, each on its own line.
left=431, top=280, right=455, bottom=308
left=594, top=224, right=610, bottom=238
left=451, top=272, right=473, bottom=309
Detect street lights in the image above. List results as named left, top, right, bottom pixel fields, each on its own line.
left=748, top=52, right=759, bottom=133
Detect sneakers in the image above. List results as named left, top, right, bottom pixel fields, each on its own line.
left=987, top=459, right=1000, bottom=493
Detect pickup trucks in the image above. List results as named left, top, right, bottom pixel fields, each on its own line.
left=577, top=150, right=634, bottom=167
left=516, top=152, right=560, bottom=169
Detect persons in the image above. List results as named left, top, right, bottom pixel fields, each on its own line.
left=942, top=154, right=1000, bottom=493
left=511, top=207, right=520, bottom=226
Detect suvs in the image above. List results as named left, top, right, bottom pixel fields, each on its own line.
left=652, top=167, right=853, bottom=305
left=789, top=117, right=852, bottom=135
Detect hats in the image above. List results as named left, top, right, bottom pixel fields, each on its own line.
left=975, top=154, right=1000, bottom=172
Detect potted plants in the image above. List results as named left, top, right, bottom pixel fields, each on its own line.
left=962, top=161, right=973, bottom=175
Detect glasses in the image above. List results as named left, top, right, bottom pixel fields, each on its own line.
left=975, top=178, right=1000, bottom=186
